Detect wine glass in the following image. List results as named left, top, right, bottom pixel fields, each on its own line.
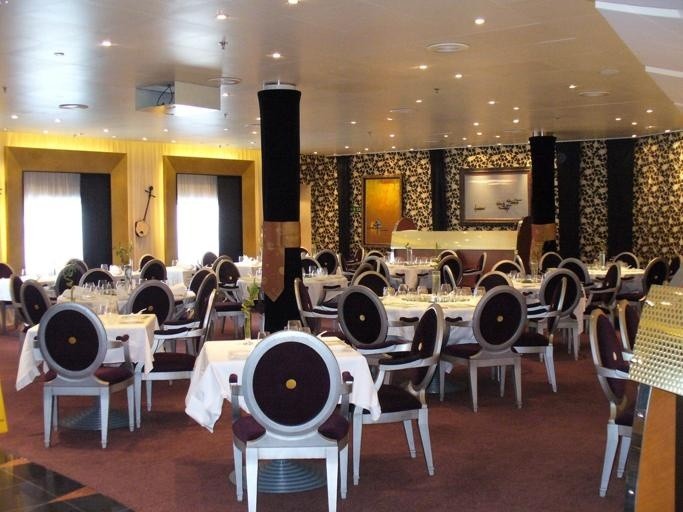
left=301, top=245, right=328, bottom=281
left=239, top=256, right=262, bottom=284
left=172, top=260, right=177, bottom=270
left=73, top=264, right=132, bottom=324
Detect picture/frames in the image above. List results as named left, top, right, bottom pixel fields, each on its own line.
left=361, top=173, right=403, bottom=248
left=459, top=167, right=532, bottom=223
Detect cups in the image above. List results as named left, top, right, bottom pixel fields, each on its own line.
left=383, top=271, right=486, bottom=306
left=258, top=320, right=312, bottom=340
left=510, top=260, right=553, bottom=287
left=386, top=247, right=432, bottom=266
left=582, top=251, right=612, bottom=272
